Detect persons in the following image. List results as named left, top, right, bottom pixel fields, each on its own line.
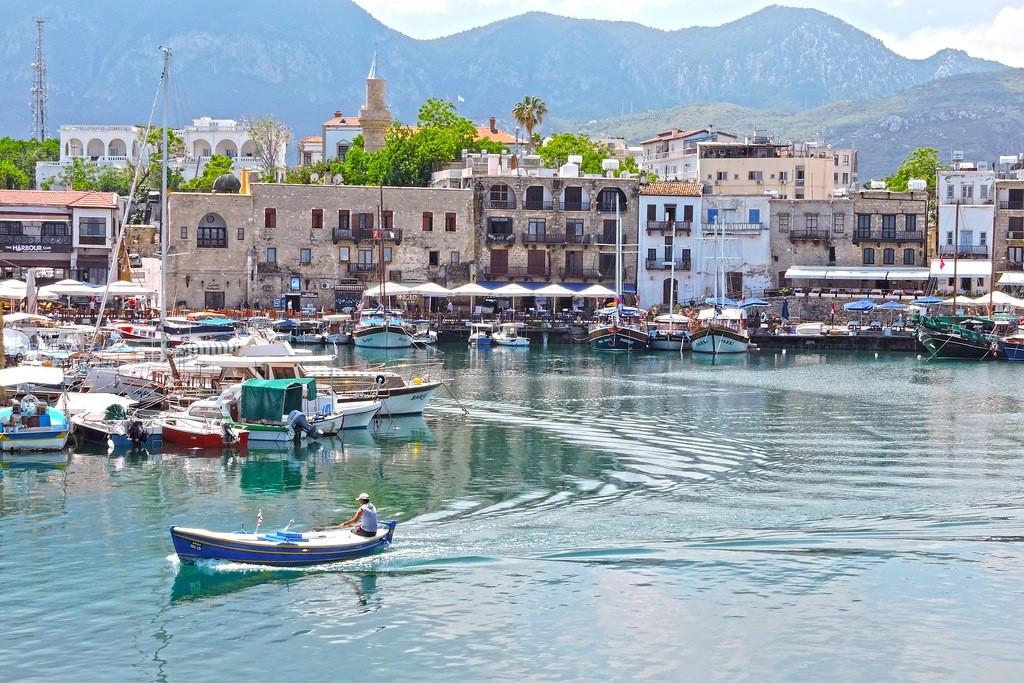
left=447, top=300, right=453, bottom=312
left=113, top=295, right=151, bottom=318
left=254, top=299, right=259, bottom=309
left=338, top=493, right=377, bottom=538
left=240, top=299, right=246, bottom=311
left=755, top=309, right=766, bottom=318
left=287, top=299, right=293, bottom=310
left=89, top=299, right=95, bottom=318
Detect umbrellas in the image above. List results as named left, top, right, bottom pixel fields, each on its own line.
left=738, top=296, right=769, bottom=315
left=974, top=291, right=1013, bottom=306
left=576, top=285, right=617, bottom=310
left=452, top=283, right=492, bottom=313
left=705, top=296, right=736, bottom=308
left=845, top=299, right=876, bottom=325
left=490, top=283, right=535, bottom=319
left=913, top=295, right=943, bottom=315
left=411, top=282, right=456, bottom=314
left=781, top=298, right=790, bottom=325
left=880, top=300, right=906, bottom=323
left=0, top=279, right=60, bottom=314
left=99, top=280, right=156, bottom=309
left=362, top=282, right=414, bottom=308
left=41, top=278, right=102, bottom=310
left=532, top=284, right=574, bottom=314
left=943, top=295, right=974, bottom=309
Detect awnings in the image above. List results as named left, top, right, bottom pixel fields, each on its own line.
left=784, top=269, right=827, bottom=279
left=826, top=270, right=888, bottom=280
left=887, top=270, right=930, bottom=280
left=930, top=260, right=992, bottom=278
left=997, top=272, right=1024, bottom=286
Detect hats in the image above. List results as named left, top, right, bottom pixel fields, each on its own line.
left=356, top=493, right=370, bottom=500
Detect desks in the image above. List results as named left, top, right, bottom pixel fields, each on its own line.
left=869, top=322, right=882, bottom=331
left=892, top=321, right=904, bottom=332
left=848, top=321, right=861, bottom=331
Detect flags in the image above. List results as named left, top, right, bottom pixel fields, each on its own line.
left=940, top=258, right=945, bottom=270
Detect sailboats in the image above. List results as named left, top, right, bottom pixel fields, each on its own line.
left=910, top=199, right=1024, bottom=363
left=588, top=188, right=756, bottom=356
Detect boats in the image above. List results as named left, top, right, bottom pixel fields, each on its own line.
left=167, top=515, right=396, bottom=572
left=1, top=47, right=451, bottom=459
left=466, top=318, right=532, bottom=350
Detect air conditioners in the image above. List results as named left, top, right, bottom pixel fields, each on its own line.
left=715, top=180, right=720, bottom=185
left=779, top=180, right=786, bottom=184
left=757, top=180, right=763, bottom=184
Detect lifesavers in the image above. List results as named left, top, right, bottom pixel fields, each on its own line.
left=1019, top=316, right=1024, bottom=325
left=21, top=395, right=39, bottom=412
left=652, top=307, right=657, bottom=314
left=685, top=308, right=691, bottom=317
left=376, top=375, right=385, bottom=384
left=641, top=311, right=647, bottom=318
left=742, top=295, right=746, bottom=303
